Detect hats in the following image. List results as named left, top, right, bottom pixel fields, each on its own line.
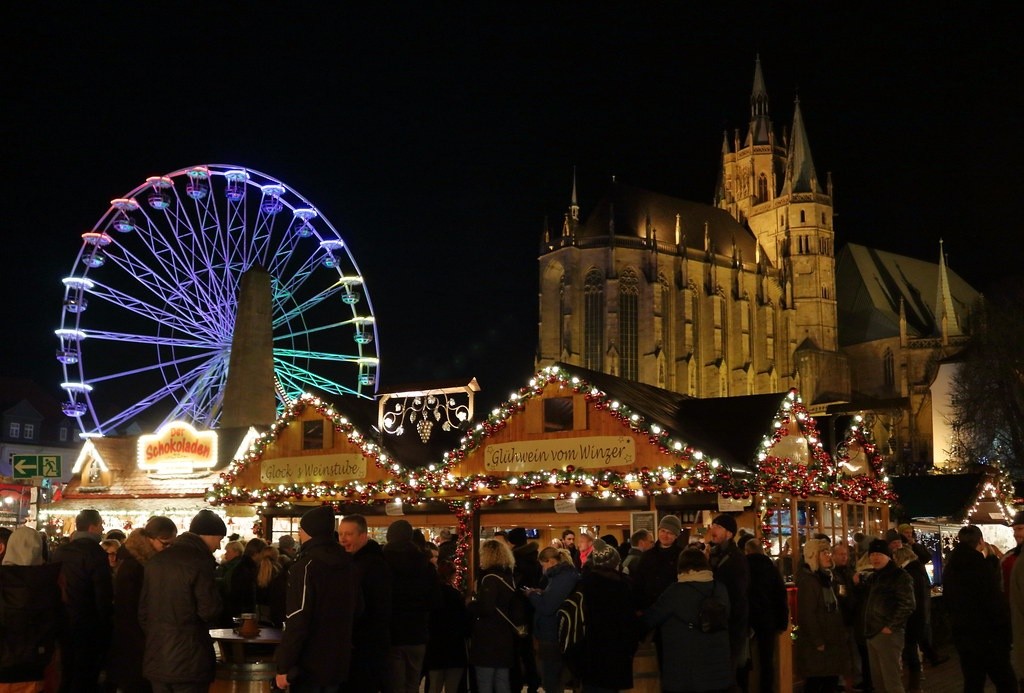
left=189, top=511, right=227, bottom=537
left=591, top=539, right=621, bottom=569
left=868, top=540, right=892, bottom=558
left=658, top=515, right=681, bottom=538
left=508, top=528, right=528, bottom=547
left=713, top=514, right=737, bottom=535
left=386, top=519, right=414, bottom=543
left=1009, top=511, right=1024, bottom=527
left=299, top=506, right=336, bottom=538
left=804, top=537, right=836, bottom=572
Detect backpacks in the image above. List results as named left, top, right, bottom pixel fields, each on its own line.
left=682, top=579, right=725, bottom=635
left=481, top=573, right=529, bottom=637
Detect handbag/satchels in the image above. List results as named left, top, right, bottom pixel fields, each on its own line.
left=555, top=591, right=585, bottom=655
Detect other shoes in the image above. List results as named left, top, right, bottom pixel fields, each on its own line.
left=932, top=654, right=951, bottom=668
left=853, top=681, right=864, bottom=690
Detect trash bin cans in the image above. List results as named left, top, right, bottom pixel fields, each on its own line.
left=621, top=630, right=662, bottom=693
left=209, top=627, right=282, bottom=693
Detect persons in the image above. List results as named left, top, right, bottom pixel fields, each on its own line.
left=275, top=507, right=362, bottom=693
left=583, top=538, right=640, bottom=693
left=465, top=541, right=521, bottom=693
left=648, top=547, right=732, bottom=693
left=942, top=526, right=1019, bottom=693
left=381, top=521, right=436, bottom=693
left=524, top=546, right=579, bottom=693
left=0, top=487, right=1024, bottom=693
left=854, top=538, right=917, bottom=693
left=796, top=538, right=852, bottom=693
left=143, top=510, right=226, bottom=693
left=53, top=510, right=113, bottom=692
